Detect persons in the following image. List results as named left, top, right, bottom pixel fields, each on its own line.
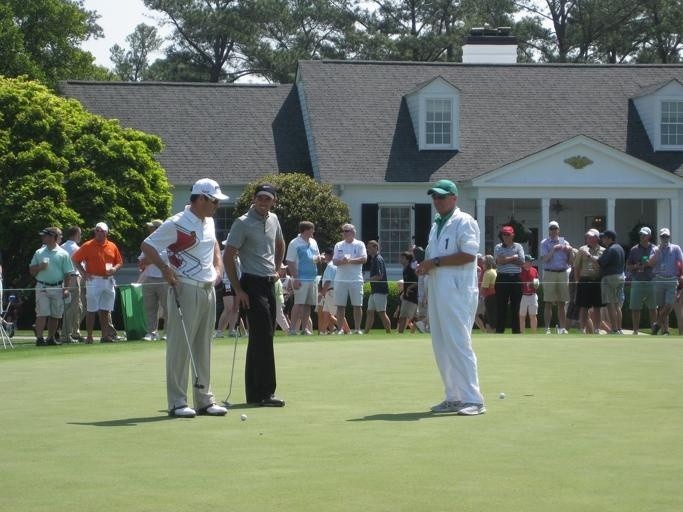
left=272, top=221, right=428, bottom=334
left=26, top=227, right=74, bottom=346
left=136, top=219, right=169, bottom=343
left=217, top=240, right=241, bottom=338
left=39, top=226, right=68, bottom=343
left=136, top=176, right=229, bottom=418
left=0, top=264, right=15, bottom=338
left=222, top=181, right=289, bottom=407
left=473, top=220, right=683, bottom=336
left=69, top=222, right=123, bottom=343
left=411, top=178, right=487, bottom=417
left=59, top=227, right=89, bottom=344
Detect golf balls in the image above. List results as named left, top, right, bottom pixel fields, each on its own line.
left=240, top=414, right=246, bottom=421
left=500, top=393, right=505, bottom=398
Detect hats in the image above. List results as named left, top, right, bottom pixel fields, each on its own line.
left=660, top=228, right=670, bottom=235
left=548, top=220, right=559, bottom=228
left=255, top=183, right=276, bottom=201
left=502, top=226, right=513, bottom=234
left=96, top=222, right=109, bottom=232
left=640, top=226, right=651, bottom=236
left=145, top=219, right=162, bottom=227
left=585, top=229, right=616, bottom=239
left=38, top=228, right=57, bottom=236
left=191, top=178, right=230, bottom=201
left=525, top=254, right=535, bottom=262
left=427, top=180, right=458, bottom=196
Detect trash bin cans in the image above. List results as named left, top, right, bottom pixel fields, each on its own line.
left=119, top=283, right=147, bottom=340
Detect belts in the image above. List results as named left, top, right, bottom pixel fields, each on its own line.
left=544, top=268, right=564, bottom=273
left=245, top=273, right=274, bottom=281
left=178, top=278, right=214, bottom=288
left=91, top=275, right=113, bottom=278
left=36, top=280, right=62, bottom=286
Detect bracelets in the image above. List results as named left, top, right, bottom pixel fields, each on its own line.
left=158, top=265, right=168, bottom=272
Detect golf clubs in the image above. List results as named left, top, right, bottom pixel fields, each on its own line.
left=172, top=283, right=204, bottom=389
left=222, top=303, right=241, bottom=406
left=63, top=297, right=69, bottom=344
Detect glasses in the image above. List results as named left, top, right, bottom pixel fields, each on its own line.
left=431, top=193, right=446, bottom=199
left=341, top=230, right=350, bottom=232
left=198, top=189, right=219, bottom=203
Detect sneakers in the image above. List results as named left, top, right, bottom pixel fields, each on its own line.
left=246, top=394, right=285, bottom=407
left=143, top=332, right=167, bottom=342
left=215, top=329, right=248, bottom=338
left=634, top=322, right=669, bottom=336
left=414, top=321, right=426, bottom=333
left=168, top=405, right=196, bottom=417
left=458, top=403, right=485, bottom=415
left=35, top=333, right=115, bottom=345
left=431, top=401, right=462, bottom=412
left=286, top=328, right=363, bottom=335
left=580, top=327, right=624, bottom=335
left=545, top=327, right=569, bottom=334
left=196, top=402, right=227, bottom=415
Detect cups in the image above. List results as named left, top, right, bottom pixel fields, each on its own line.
left=43, top=258, right=49, bottom=266
left=344, top=253, right=351, bottom=263
left=313, top=255, right=318, bottom=263
left=105, top=263, right=113, bottom=275
left=637, top=263, right=645, bottom=273
